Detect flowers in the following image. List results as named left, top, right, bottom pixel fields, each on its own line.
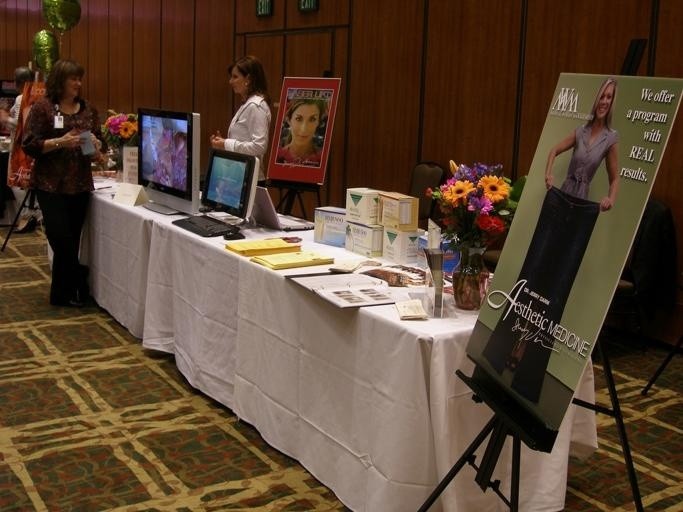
left=423, top=158, right=528, bottom=309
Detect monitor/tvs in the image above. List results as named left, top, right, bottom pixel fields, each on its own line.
left=137, top=107, right=201, bottom=214
left=201, top=149, right=260, bottom=219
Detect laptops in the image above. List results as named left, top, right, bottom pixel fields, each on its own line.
left=253, top=186, right=315, bottom=230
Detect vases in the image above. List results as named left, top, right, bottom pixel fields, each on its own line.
left=115, top=145, right=125, bottom=182
left=451, top=247, right=490, bottom=309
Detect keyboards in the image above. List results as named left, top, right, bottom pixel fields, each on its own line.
left=172, top=216, right=240, bottom=237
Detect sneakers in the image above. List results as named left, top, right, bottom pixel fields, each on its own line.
left=504, top=355, right=519, bottom=371
left=49, top=298, right=85, bottom=307
left=74, top=262, right=91, bottom=304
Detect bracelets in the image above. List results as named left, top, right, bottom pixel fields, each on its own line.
left=54, top=138, right=61, bottom=150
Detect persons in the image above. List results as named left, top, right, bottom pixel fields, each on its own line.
left=276, top=96, right=330, bottom=168
left=209, top=55, right=272, bottom=187
left=503, top=78, right=620, bottom=373
left=0, top=67, right=43, bottom=234
left=19, top=60, right=108, bottom=308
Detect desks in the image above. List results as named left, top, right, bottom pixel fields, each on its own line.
left=100, top=109, right=138, bottom=147
left=78, top=176, right=584, bottom=512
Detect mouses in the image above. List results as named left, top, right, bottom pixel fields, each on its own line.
left=224, top=232, right=245, bottom=240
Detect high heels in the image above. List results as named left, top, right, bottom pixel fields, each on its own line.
left=9, top=214, right=36, bottom=233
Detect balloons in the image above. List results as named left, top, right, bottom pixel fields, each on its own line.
left=42, top=0, right=80, bottom=33
left=33, top=29, right=59, bottom=73
